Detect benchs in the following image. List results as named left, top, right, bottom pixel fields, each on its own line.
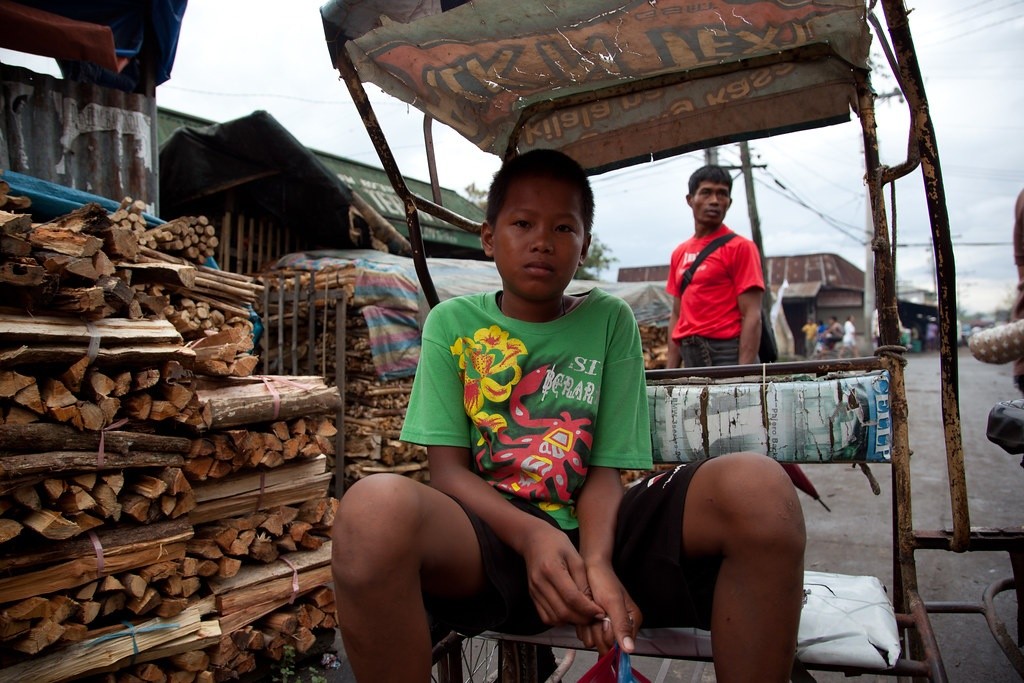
left=448, top=353, right=932, bottom=676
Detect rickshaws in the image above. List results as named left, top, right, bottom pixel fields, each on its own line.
left=320, top=0, right=1023, bottom=683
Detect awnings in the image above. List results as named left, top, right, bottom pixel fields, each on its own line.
left=817, top=291, right=862, bottom=307
left=775, top=281, right=822, bottom=297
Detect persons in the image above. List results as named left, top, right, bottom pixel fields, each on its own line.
left=911, top=313, right=939, bottom=353
left=802, top=315, right=858, bottom=360
left=665, top=165, right=765, bottom=370
left=331, top=148, right=807, bottom=683
left=1013, top=188, right=1024, bottom=398
